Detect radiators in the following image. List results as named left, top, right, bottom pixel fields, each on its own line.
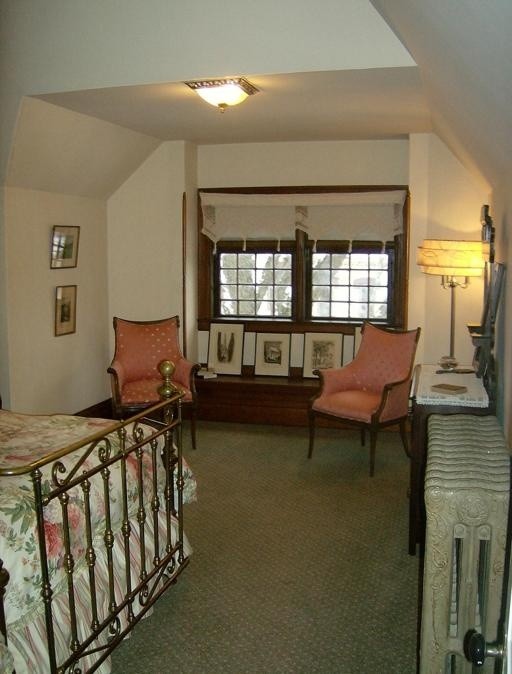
left=419, top=413, right=510, bottom=660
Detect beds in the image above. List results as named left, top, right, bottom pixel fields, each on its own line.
left=0, top=359, right=199, bottom=674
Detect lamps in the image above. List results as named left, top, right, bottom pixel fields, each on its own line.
left=183, top=77, right=261, bottom=112
left=416, top=238, right=486, bottom=357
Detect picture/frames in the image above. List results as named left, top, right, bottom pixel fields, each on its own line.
left=205, top=322, right=344, bottom=381
left=50, top=225, right=80, bottom=337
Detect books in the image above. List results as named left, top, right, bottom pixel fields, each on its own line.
left=430, top=382, right=467, bottom=394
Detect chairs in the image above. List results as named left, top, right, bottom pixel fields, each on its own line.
left=106, top=315, right=203, bottom=450
left=306, top=319, right=422, bottom=477
